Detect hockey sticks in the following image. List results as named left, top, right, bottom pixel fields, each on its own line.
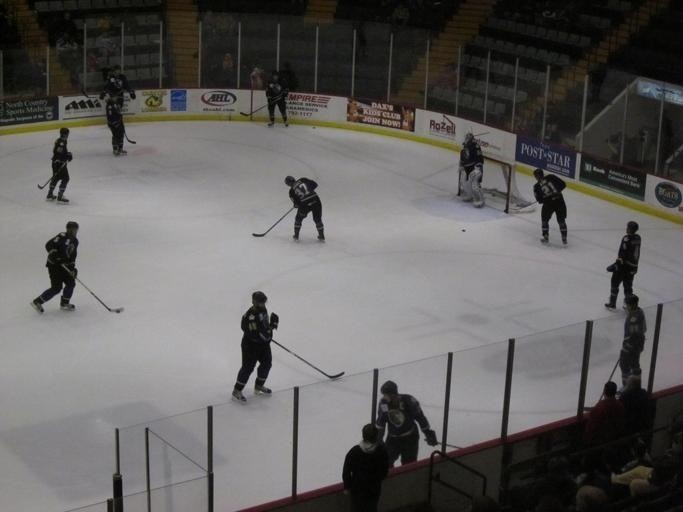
left=252, top=207, right=295, bottom=236
left=38, top=158, right=69, bottom=189
left=583, top=357, right=620, bottom=411
left=271, top=340, right=345, bottom=378
left=62, top=265, right=124, bottom=313
left=510, top=201, right=538, bottom=211
left=125, top=132, right=136, bottom=143
left=240, top=97, right=282, bottom=116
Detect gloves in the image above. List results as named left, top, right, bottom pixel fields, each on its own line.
left=607, top=259, right=622, bottom=272
left=269, top=312, right=278, bottom=328
left=130, top=90, right=135, bottom=99
left=100, top=92, right=106, bottom=99
left=426, top=431, right=437, bottom=446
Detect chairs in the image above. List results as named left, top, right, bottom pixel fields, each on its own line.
left=201, top=2, right=448, bottom=109
left=430, top=2, right=683, bottom=135
left=524, top=423, right=682, bottom=510
left=1, top=3, right=172, bottom=87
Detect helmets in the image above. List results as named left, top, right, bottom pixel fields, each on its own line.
left=252, top=292, right=267, bottom=312
left=285, top=176, right=295, bottom=187
left=534, top=169, right=543, bottom=179
left=623, top=294, right=638, bottom=315
left=627, top=222, right=638, bottom=234
left=381, top=381, right=398, bottom=401
left=60, top=128, right=69, bottom=138
left=67, top=222, right=78, bottom=240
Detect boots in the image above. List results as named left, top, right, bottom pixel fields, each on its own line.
left=605, top=295, right=617, bottom=308
left=318, top=228, right=324, bottom=239
left=118, top=148, right=127, bottom=154
left=61, top=296, right=74, bottom=308
left=57, top=192, right=69, bottom=202
left=33, top=296, right=44, bottom=312
left=113, top=146, right=118, bottom=153
left=293, top=231, right=299, bottom=239
left=47, top=189, right=56, bottom=199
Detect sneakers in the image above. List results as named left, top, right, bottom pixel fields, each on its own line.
left=255, top=384, right=271, bottom=393
left=232, top=388, right=246, bottom=401
left=540, top=239, right=549, bottom=244
left=285, top=121, right=288, bottom=126
left=268, top=120, right=274, bottom=126
left=462, top=195, right=484, bottom=207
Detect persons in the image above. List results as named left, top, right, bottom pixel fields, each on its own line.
left=338, top=380, right=439, bottom=511
left=587, top=221, right=654, bottom=446
left=96, top=65, right=137, bottom=156
left=470, top=431, right=682, bottom=511
left=230, top=292, right=278, bottom=405
left=400, top=103, right=414, bottom=130
left=283, top=176, right=326, bottom=241
left=532, top=166, right=568, bottom=250
left=45, top=128, right=73, bottom=202
left=263, top=69, right=290, bottom=128
left=348, top=98, right=363, bottom=122
left=30, top=221, right=78, bottom=313
left=458, top=133, right=486, bottom=207
